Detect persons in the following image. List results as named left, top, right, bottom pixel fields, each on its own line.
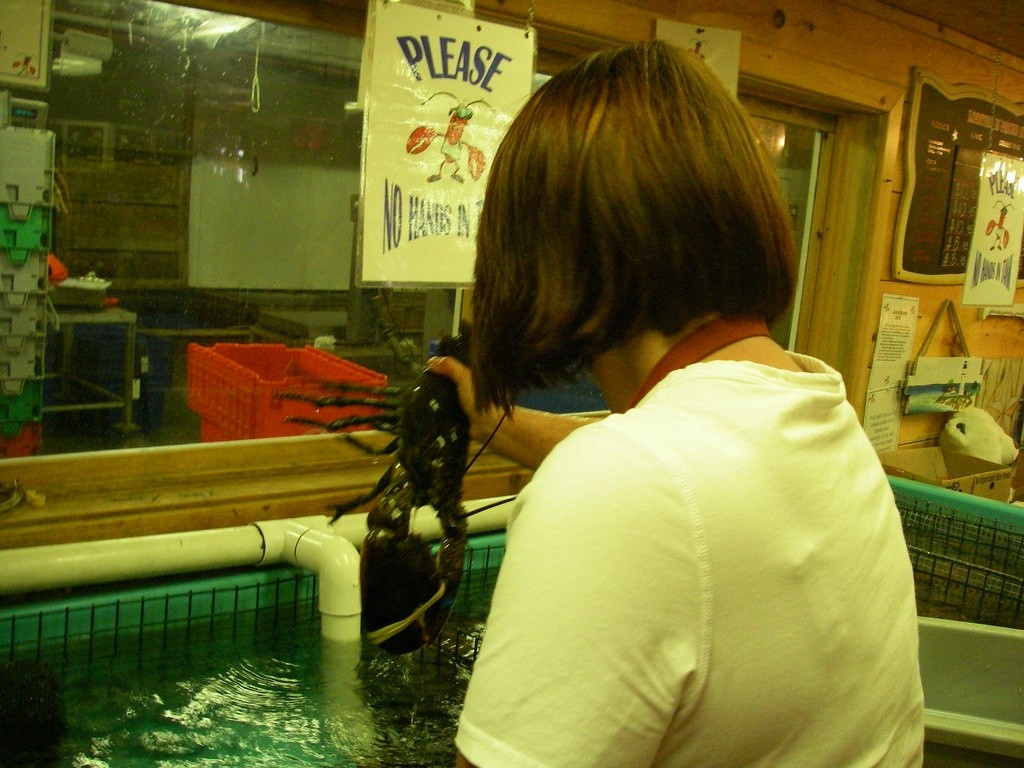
left=427, top=42, right=924, bottom=767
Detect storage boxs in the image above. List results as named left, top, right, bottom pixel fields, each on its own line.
left=0, top=125, right=57, bottom=459
left=184, top=339, right=389, bottom=443
left=879, top=447, right=1014, bottom=503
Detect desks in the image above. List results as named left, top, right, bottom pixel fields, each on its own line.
left=43, top=307, right=138, bottom=448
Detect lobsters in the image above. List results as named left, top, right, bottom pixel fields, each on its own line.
left=273, top=287, right=517, bottom=652
